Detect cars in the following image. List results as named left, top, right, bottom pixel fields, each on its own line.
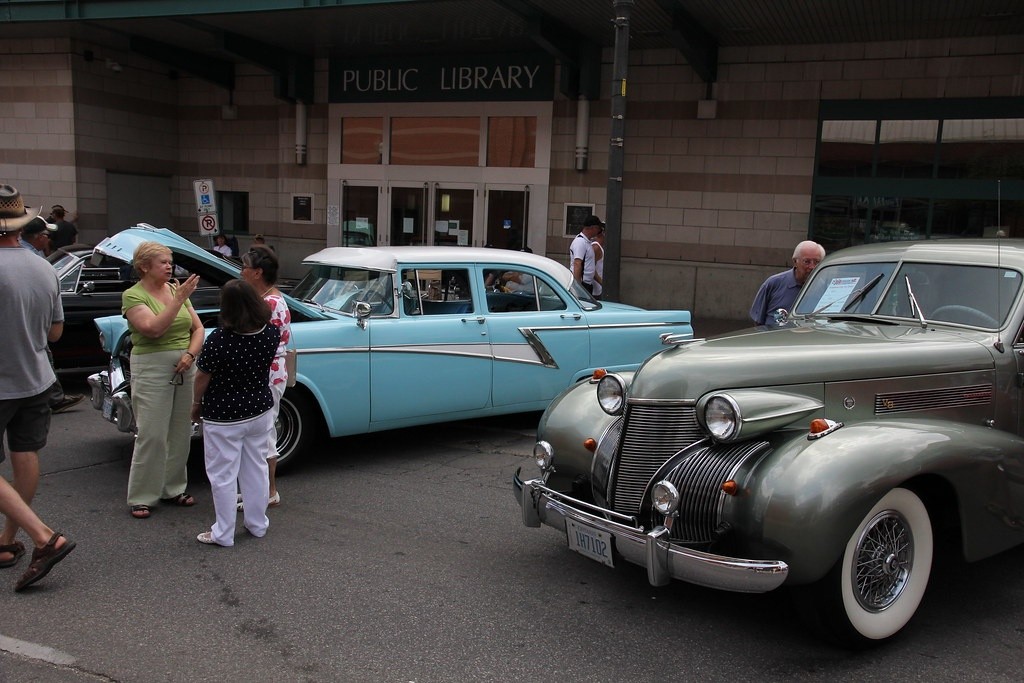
left=48, top=245, right=315, bottom=382
left=88, top=223, right=693, bottom=477
left=512, top=231, right=1024, bottom=645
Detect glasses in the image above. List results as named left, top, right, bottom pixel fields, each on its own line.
left=241, top=265, right=258, bottom=271
left=169, top=364, right=184, bottom=385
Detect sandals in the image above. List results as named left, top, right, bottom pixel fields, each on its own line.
left=159, top=492, right=195, bottom=506
left=132, top=505, right=151, bottom=519
left=0, top=540, right=26, bottom=569
left=14, top=531, right=76, bottom=593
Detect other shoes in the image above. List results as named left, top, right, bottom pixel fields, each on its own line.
left=236, top=491, right=281, bottom=510
left=50, top=393, right=86, bottom=414
left=197, top=531, right=217, bottom=543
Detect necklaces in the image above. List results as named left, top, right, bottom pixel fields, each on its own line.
left=260, top=286, right=273, bottom=298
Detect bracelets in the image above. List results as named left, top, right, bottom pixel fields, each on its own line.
left=193, top=400, right=200, bottom=406
left=186, top=352, right=195, bottom=362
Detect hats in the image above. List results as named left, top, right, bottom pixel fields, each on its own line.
left=23, top=215, right=58, bottom=234
left=583, top=215, right=605, bottom=227
left=254, top=234, right=264, bottom=239
left=0, top=183, right=43, bottom=232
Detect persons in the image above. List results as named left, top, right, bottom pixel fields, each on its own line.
left=236, top=251, right=291, bottom=512
left=569, top=216, right=605, bottom=301
left=254, top=234, right=275, bottom=252
left=0, top=184, right=76, bottom=593
left=121, top=242, right=205, bottom=519
left=213, top=234, right=232, bottom=260
left=483, top=244, right=555, bottom=296
left=249, top=243, right=279, bottom=270
left=749, top=239, right=826, bottom=328
left=190, top=278, right=281, bottom=547
left=18, top=216, right=86, bottom=414
left=45, top=205, right=80, bottom=257
